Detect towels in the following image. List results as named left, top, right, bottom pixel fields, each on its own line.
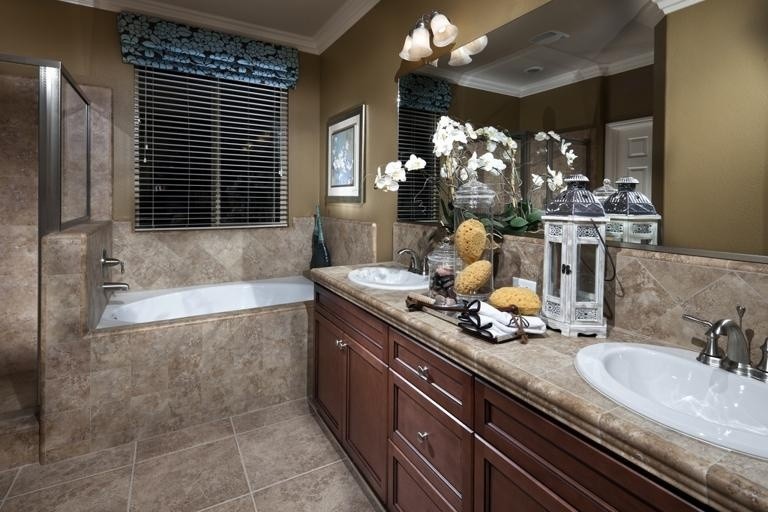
left=463, top=300, right=546, bottom=339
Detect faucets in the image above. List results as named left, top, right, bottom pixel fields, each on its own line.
left=705, top=318, right=750, bottom=364
left=102, top=282, right=130, bottom=292
left=397, top=248, right=419, bottom=271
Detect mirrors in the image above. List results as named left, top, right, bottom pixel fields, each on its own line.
left=396, top=1, right=767, bottom=265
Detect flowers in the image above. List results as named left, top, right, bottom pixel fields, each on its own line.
left=374, top=116, right=518, bottom=239
left=435, top=127, right=577, bottom=229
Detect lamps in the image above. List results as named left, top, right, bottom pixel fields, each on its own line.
left=538, top=173, right=609, bottom=339
left=427, top=35, right=490, bottom=67
left=606, top=177, right=661, bottom=247
left=398, top=11, right=459, bottom=63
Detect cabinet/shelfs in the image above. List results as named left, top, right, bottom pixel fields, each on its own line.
left=387, top=326, right=698, bottom=512
left=311, top=307, right=385, bottom=502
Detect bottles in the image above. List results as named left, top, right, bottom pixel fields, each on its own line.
left=426, top=171, right=495, bottom=304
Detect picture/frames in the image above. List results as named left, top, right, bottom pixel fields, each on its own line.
left=323, top=104, right=366, bottom=207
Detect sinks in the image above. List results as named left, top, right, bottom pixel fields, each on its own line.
left=574, top=343, right=768, bottom=461
left=348, top=265, right=428, bottom=290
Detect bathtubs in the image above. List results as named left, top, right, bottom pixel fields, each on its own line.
left=96, top=277, right=315, bottom=330
